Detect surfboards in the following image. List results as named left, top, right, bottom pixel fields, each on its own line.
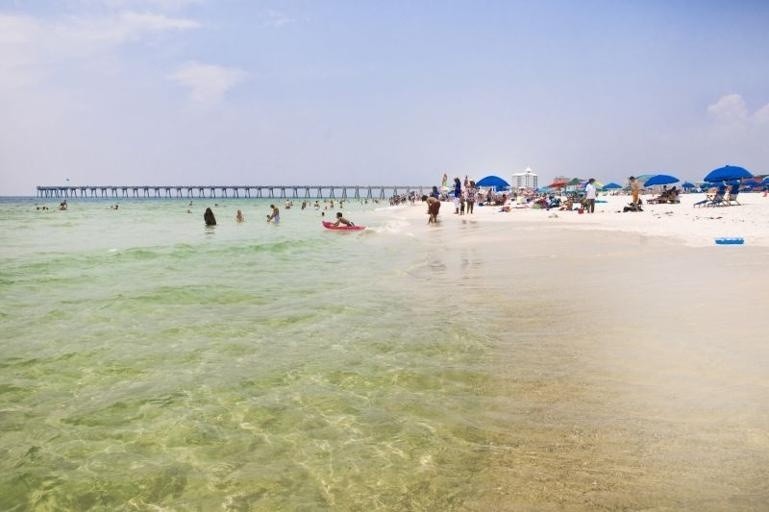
left=716, top=239, right=743, bottom=244
left=323, top=222, right=365, bottom=229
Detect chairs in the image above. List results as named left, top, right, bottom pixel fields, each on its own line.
left=693, top=190, right=741, bottom=208
left=646, top=189, right=681, bottom=204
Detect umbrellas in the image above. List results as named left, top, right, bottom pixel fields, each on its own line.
left=703, top=164, right=753, bottom=182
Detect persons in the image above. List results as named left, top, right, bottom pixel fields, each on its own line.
left=653, top=184, right=679, bottom=203
left=629, top=176, right=641, bottom=210
left=711, top=181, right=740, bottom=202
left=389, top=174, right=597, bottom=223
left=266, top=198, right=380, bottom=227
left=235, top=210, right=244, bottom=221
left=186, top=200, right=218, bottom=213
left=110, top=205, right=119, bottom=210
left=36, top=199, right=68, bottom=213
